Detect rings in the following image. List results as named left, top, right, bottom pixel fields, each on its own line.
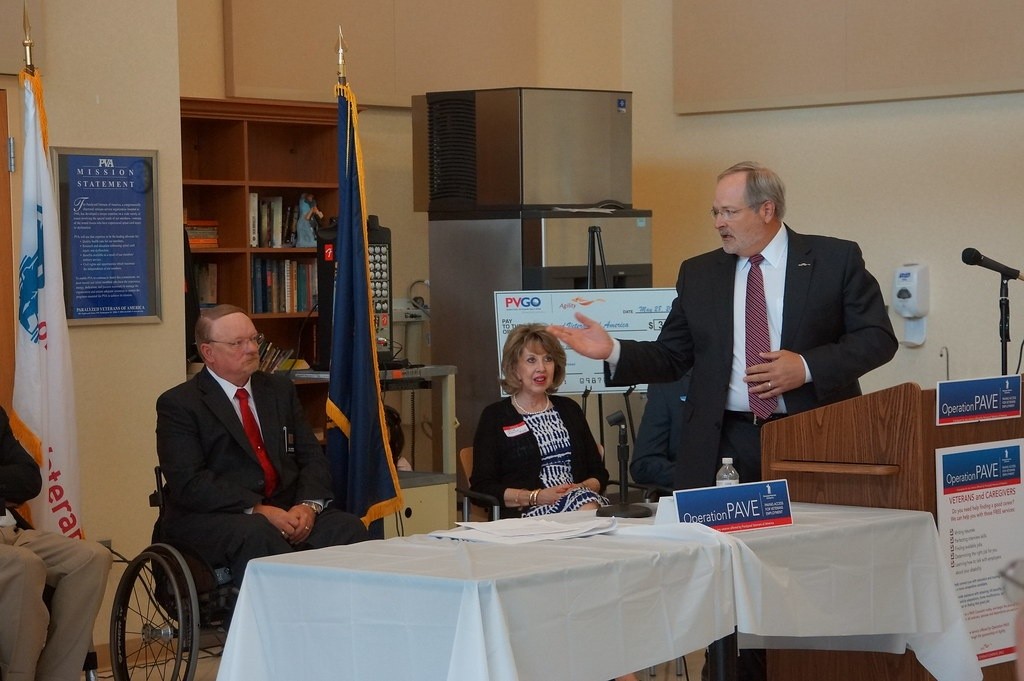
left=305, top=526, right=310, bottom=531
left=280, top=531, right=286, bottom=537
left=769, top=382, right=772, bottom=389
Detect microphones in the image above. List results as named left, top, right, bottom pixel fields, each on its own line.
left=962, top=248, right=1024, bottom=280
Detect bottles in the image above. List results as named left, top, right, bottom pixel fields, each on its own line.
left=716, top=458, right=739, bottom=487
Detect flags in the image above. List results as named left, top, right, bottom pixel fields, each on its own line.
left=10, top=68, right=83, bottom=546
left=324, top=83, right=405, bottom=541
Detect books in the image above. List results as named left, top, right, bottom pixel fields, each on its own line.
left=249, top=193, right=299, bottom=249
left=191, top=263, right=218, bottom=308
left=258, top=339, right=311, bottom=374
left=252, top=255, right=317, bottom=314
left=183, top=219, right=221, bottom=249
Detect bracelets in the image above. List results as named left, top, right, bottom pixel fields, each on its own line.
left=515, top=488, right=525, bottom=506
left=528, top=489, right=542, bottom=506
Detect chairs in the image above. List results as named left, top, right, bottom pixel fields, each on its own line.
left=457, top=445, right=607, bottom=523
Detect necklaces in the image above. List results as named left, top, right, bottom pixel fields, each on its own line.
left=514, top=394, right=549, bottom=414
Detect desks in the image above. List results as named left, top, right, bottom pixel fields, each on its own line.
left=214, top=499, right=934, bottom=681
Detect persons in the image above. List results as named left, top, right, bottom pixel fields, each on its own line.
left=0, top=404, right=112, bottom=681
left=155, top=305, right=371, bottom=633
left=297, top=192, right=323, bottom=248
left=546, top=162, right=898, bottom=681
left=629, top=369, right=690, bottom=499
left=469, top=323, right=611, bottom=518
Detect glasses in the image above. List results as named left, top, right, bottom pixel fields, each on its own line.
left=208, top=332, right=264, bottom=347
left=710, top=200, right=770, bottom=220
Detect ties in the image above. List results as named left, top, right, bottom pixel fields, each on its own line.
left=746, top=253, right=777, bottom=421
left=234, top=389, right=277, bottom=498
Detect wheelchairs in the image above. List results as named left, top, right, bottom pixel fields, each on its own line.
left=107, top=464, right=241, bottom=681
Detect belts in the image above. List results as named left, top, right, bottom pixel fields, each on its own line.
left=723, top=409, right=789, bottom=427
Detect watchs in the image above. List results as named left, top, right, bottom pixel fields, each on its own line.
left=300, top=501, right=321, bottom=515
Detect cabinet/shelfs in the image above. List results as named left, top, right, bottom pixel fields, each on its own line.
left=180, top=96, right=337, bottom=370
